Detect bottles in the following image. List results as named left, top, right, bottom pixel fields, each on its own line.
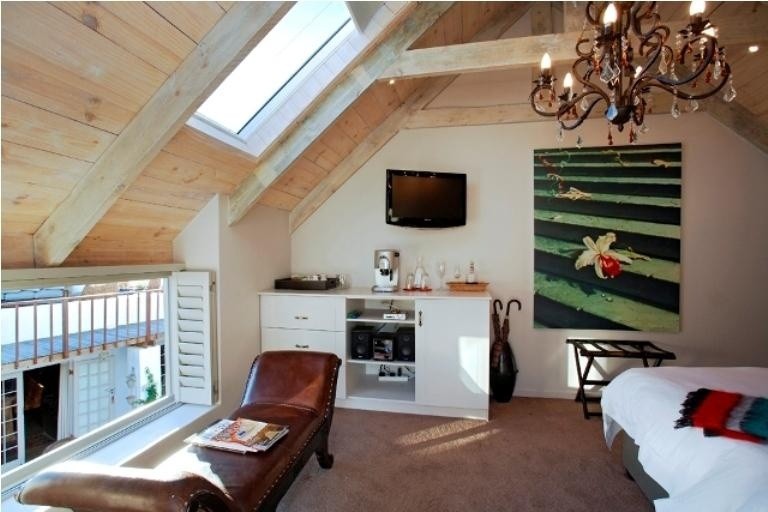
left=415, top=257, right=425, bottom=287
left=453, top=260, right=478, bottom=282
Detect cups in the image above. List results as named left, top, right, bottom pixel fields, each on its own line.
left=405, top=274, right=415, bottom=288
left=338, top=272, right=351, bottom=289
left=421, top=273, right=430, bottom=289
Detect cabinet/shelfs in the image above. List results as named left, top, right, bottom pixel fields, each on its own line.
left=257, top=286, right=493, bottom=422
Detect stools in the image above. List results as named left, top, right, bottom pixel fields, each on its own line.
left=565, top=339, right=675, bottom=420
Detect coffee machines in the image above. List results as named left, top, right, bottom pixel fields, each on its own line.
left=371, top=249, right=400, bottom=293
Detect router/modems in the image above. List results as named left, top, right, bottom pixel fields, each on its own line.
left=383, top=311, right=408, bottom=321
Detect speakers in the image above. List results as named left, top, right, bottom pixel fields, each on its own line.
left=395, top=326, right=414, bottom=361
left=350, top=325, right=376, bottom=360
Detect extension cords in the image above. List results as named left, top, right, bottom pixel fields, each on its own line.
left=378, top=374, right=409, bottom=382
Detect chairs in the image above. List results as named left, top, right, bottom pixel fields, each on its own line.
left=12, top=351, right=340, bottom=512
left=12, top=372, right=47, bottom=431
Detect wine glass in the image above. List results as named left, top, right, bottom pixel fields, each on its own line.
left=436, top=262, right=446, bottom=291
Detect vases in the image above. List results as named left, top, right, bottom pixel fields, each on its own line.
left=490, top=340, right=517, bottom=402
left=555, top=231, right=633, bottom=281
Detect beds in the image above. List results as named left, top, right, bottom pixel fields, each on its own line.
left=600, top=365, right=768, bottom=512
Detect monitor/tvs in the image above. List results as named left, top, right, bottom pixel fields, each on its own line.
left=385, top=169, right=467, bottom=228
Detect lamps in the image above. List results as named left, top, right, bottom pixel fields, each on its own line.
left=527, top=1, right=736, bottom=150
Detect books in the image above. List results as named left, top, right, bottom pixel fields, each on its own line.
left=183, top=419, right=289, bottom=454
